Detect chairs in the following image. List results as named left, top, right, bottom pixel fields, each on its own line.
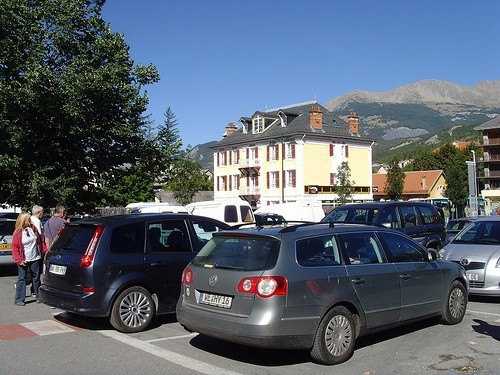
left=404, top=215, right=416, bottom=227
left=149, top=227, right=187, bottom=251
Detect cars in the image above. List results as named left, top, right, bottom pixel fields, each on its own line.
left=438, top=216, right=500, bottom=297
left=38, top=213, right=231, bottom=333
left=0, top=218, right=17, bottom=266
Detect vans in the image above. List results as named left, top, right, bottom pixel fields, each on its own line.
left=186, top=200, right=256, bottom=228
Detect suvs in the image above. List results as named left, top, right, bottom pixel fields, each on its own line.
left=176, top=221, right=470, bottom=366
left=320, top=201, right=446, bottom=261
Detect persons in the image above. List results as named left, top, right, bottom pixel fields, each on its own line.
left=12, top=212, right=48, bottom=306
left=478, top=207, right=497, bottom=216
left=14, top=205, right=43, bottom=297
left=44, top=205, right=69, bottom=248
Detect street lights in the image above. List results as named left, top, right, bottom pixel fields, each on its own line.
left=269, top=134, right=299, bottom=204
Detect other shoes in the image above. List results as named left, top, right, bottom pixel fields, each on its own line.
left=37, top=298, right=41, bottom=303
left=16, top=300, right=25, bottom=306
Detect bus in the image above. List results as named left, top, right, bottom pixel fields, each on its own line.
left=408, top=197, right=488, bottom=224
